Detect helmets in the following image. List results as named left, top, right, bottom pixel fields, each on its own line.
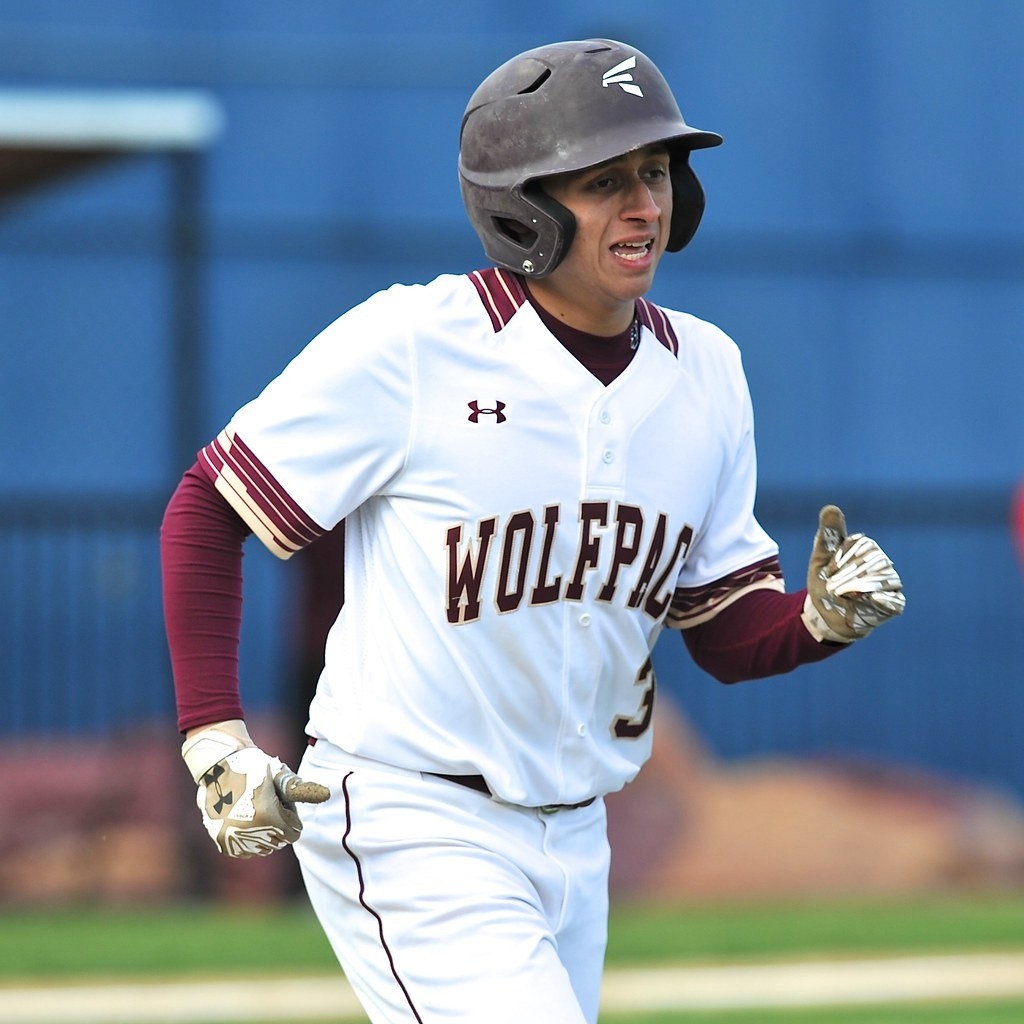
left=457, top=37, right=724, bottom=279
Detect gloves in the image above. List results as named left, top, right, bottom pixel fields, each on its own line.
left=799, top=504, right=906, bottom=644
left=182, top=719, right=331, bottom=861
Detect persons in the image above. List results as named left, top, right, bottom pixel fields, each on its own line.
left=161, top=40, right=906, bottom=1024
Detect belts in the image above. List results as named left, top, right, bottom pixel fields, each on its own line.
left=425, top=771, right=596, bottom=814
